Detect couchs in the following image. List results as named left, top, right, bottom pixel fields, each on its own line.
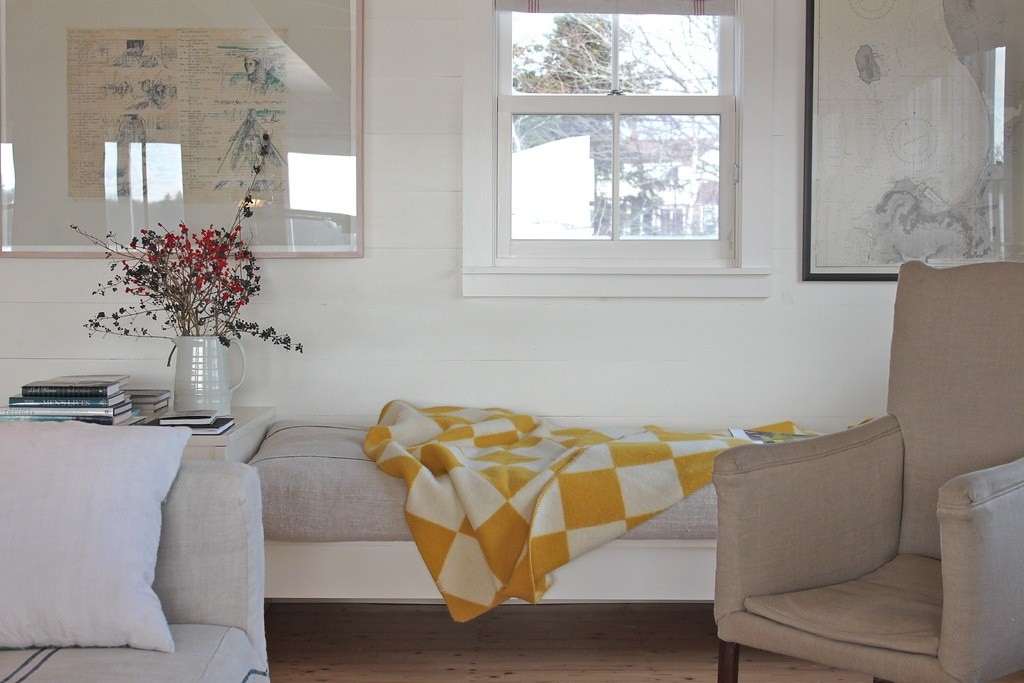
left=0, top=461, right=272, bottom=683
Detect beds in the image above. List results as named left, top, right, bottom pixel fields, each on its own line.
left=171, top=336, right=876, bottom=605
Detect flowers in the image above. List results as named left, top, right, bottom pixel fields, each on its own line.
left=70, top=131, right=304, bottom=366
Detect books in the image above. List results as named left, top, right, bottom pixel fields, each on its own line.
left=0, top=375, right=235, bottom=436
left=728, top=428, right=818, bottom=445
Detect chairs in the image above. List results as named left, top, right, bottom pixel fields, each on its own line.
left=713, top=259, right=1024, bottom=683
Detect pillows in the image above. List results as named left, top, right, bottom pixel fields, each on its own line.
left=0, top=420, right=193, bottom=652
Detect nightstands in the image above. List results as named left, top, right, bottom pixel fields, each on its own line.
left=174, top=407, right=278, bottom=465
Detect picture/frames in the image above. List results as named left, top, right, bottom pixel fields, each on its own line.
left=803, top=1, right=1024, bottom=280
left=0, top=0, right=364, bottom=259
